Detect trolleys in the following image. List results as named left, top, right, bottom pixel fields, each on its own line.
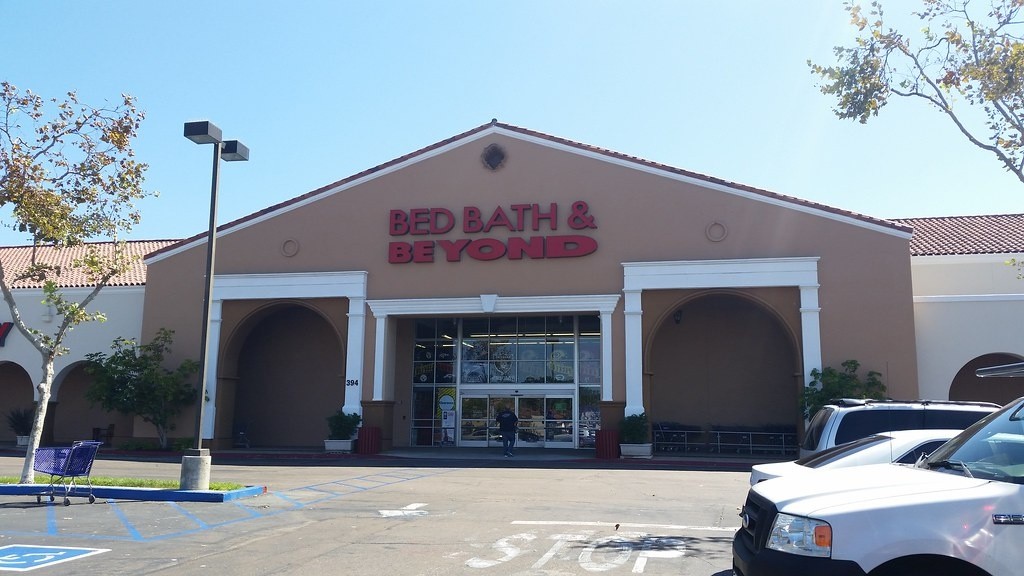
left=33, top=439, right=105, bottom=507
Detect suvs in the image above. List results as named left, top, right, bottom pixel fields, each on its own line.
left=799, top=397, right=1005, bottom=460
left=731, top=396, right=1024, bottom=576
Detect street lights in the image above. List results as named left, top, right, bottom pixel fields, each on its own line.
left=179, top=119, right=251, bottom=493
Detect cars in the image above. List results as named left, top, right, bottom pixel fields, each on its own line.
left=749, top=429, right=1024, bottom=488
left=554, top=422, right=596, bottom=447
left=462, top=428, right=540, bottom=443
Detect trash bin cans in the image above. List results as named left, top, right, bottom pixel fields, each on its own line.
left=416, top=424, right=433, bottom=445
left=358, top=427, right=379, bottom=454
left=595, top=429, right=619, bottom=459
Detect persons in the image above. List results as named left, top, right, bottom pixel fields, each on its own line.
left=496, top=404, right=518, bottom=458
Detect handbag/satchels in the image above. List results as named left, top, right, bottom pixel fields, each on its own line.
left=512, top=425, right=520, bottom=433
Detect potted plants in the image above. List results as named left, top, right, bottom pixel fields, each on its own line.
left=324, top=410, right=363, bottom=451
left=618, top=413, right=653, bottom=457
left=4, top=407, right=34, bottom=445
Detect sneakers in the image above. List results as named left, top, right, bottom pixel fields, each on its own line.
left=504, top=455, right=509, bottom=457
left=507, top=451, right=515, bottom=457
